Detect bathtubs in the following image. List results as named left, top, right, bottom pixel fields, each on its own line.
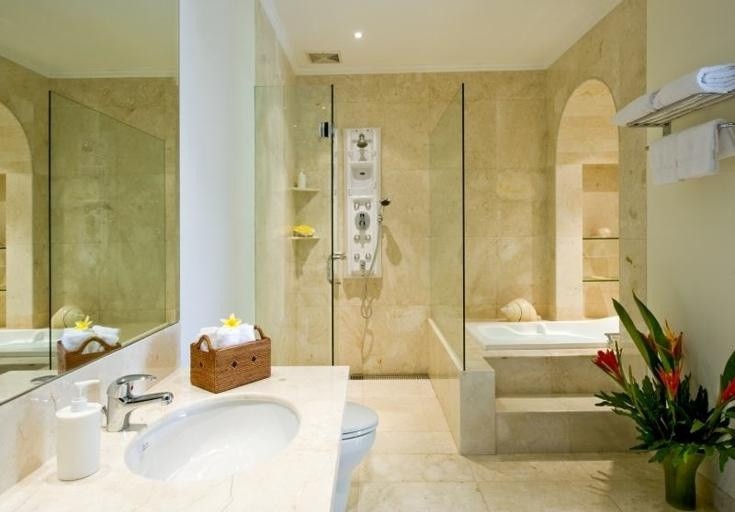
left=465, top=315, right=620, bottom=350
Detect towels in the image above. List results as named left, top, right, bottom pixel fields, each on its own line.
left=647, top=119, right=735, bottom=186
left=612, top=65, right=735, bottom=128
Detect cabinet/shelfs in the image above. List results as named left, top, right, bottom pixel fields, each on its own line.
left=290, top=185, right=320, bottom=241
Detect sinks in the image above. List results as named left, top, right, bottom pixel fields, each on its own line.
left=125, top=392, right=301, bottom=483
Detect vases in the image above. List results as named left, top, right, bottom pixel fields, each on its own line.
left=662, top=446, right=705, bottom=511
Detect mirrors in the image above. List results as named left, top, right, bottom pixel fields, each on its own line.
left=0, top=0, right=180, bottom=405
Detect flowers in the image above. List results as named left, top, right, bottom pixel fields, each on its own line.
left=588, top=289, right=735, bottom=473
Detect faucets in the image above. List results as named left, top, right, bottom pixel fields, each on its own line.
left=31, top=375, right=57, bottom=382
left=102, top=373, right=176, bottom=433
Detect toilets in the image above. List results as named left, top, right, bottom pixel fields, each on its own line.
left=330, top=401, right=379, bottom=512
